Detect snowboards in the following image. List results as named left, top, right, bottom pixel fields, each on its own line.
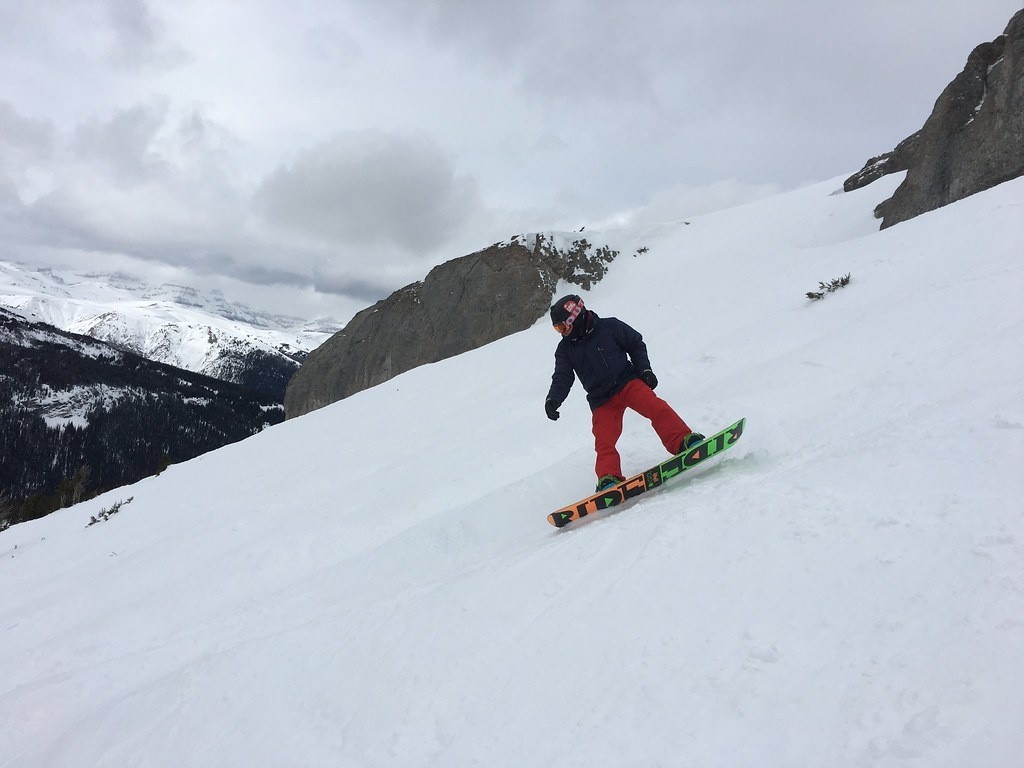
left=547, top=416, right=746, bottom=529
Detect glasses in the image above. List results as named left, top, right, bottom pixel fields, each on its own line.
left=553, top=297, right=583, bottom=336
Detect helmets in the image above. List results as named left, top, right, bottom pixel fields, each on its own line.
left=550, top=295, right=588, bottom=344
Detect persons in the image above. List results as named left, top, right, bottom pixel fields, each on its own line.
left=546, top=294, right=701, bottom=492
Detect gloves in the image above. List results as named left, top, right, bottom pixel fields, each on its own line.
left=640, top=369, right=658, bottom=390
left=545, top=398, right=561, bottom=421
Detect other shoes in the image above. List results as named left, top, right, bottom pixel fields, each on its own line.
left=595, top=474, right=621, bottom=492
left=678, top=432, right=706, bottom=453
